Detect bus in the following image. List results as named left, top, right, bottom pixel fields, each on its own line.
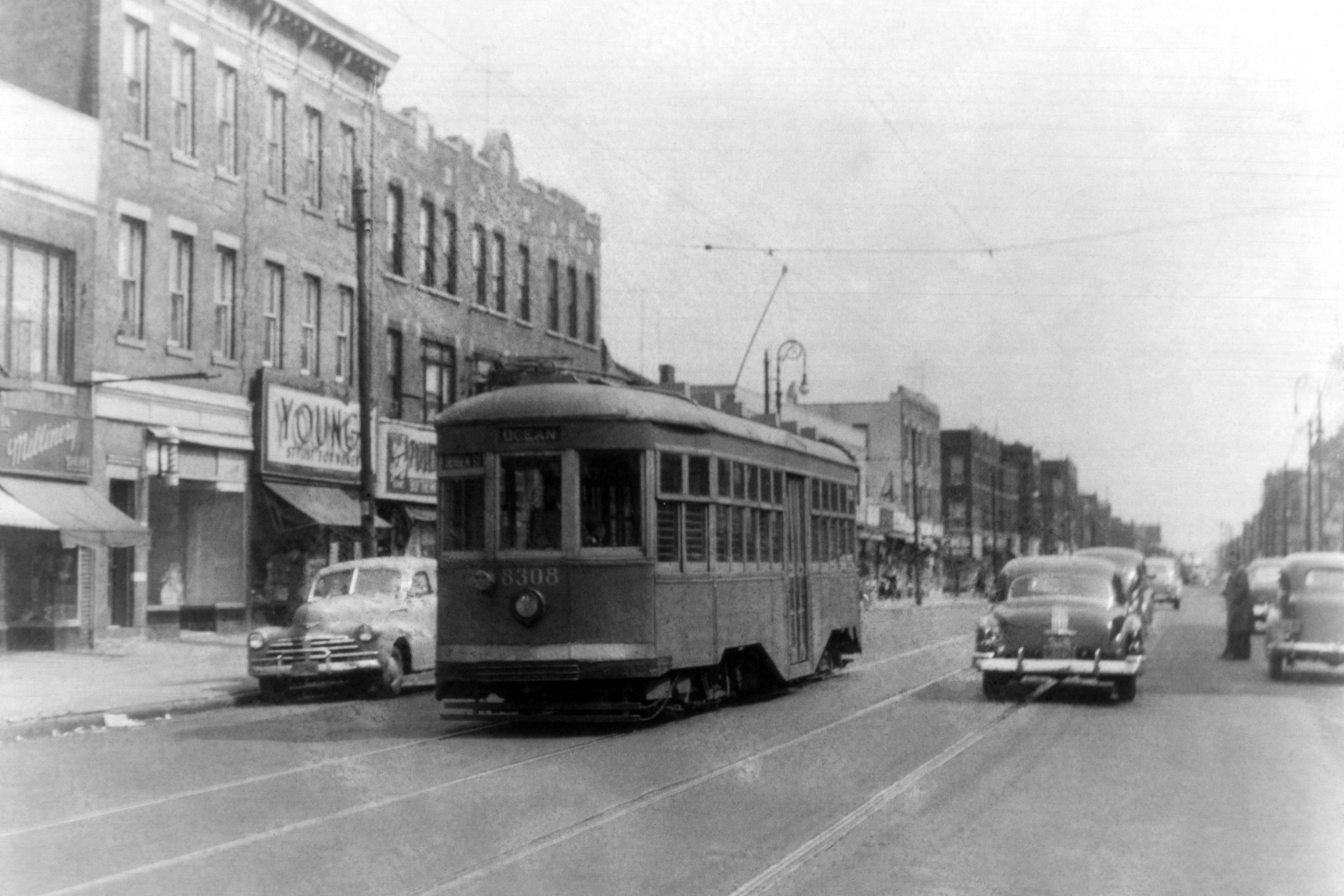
left=433, top=361, right=864, bottom=724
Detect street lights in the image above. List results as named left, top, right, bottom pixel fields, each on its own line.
left=901, top=419, right=925, bottom=604
left=775, top=339, right=810, bottom=426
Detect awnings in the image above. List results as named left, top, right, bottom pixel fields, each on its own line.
left=0, top=472, right=148, bottom=549
left=264, top=479, right=393, bottom=528
left=0, top=488, right=61, bottom=531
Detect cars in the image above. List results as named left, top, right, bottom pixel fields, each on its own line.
left=1147, top=557, right=1184, bottom=608
left=971, top=555, right=1149, bottom=700
left=246, top=556, right=437, bottom=704
left=1071, top=547, right=1155, bottom=624
left=1244, top=555, right=1288, bottom=620
left=1264, top=551, right=1344, bottom=679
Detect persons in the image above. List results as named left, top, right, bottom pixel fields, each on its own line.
left=1220, top=551, right=1254, bottom=659
left=882, top=557, right=899, bottom=599
